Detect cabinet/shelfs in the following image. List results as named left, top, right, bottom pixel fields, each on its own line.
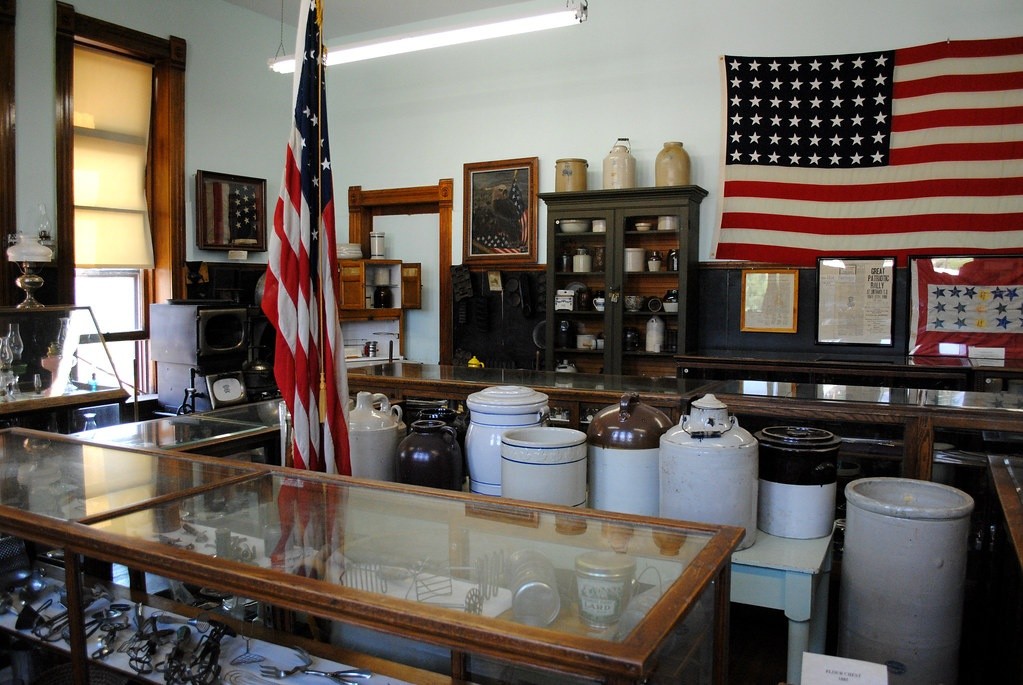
left=0, top=361, right=1023, bottom=685
left=337, top=259, right=421, bottom=370
left=536, top=184, right=708, bottom=379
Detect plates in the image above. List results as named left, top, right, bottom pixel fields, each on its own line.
left=566, top=281, right=588, bottom=313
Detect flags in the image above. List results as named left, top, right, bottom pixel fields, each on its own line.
left=709, top=35, right=1022, bottom=266
left=272, top=477, right=346, bottom=586
left=260, top=0, right=352, bottom=478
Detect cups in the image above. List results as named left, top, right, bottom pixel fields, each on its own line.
left=576, top=334, right=595, bottom=349
left=593, top=298, right=606, bottom=311
left=554, top=157, right=589, bottom=191
left=572, top=254, right=591, bottom=273
left=624, top=247, right=644, bottom=271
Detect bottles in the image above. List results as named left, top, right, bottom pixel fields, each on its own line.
left=463, top=385, right=550, bottom=495
left=645, top=317, right=664, bottom=354
left=513, top=550, right=637, bottom=629
left=602, top=137, right=637, bottom=189
left=645, top=249, right=662, bottom=272
left=345, top=392, right=468, bottom=494
left=654, top=142, right=692, bottom=186
left=559, top=248, right=586, bottom=271
left=656, top=391, right=760, bottom=553
left=661, top=289, right=679, bottom=313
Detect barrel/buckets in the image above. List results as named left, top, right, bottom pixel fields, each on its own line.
left=501, top=425, right=588, bottom=513
left=754, top=421, right=839, bottom=542
left=844, top=470, right=976, bottom=683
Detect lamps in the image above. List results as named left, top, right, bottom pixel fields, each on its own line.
left=265, top=0, right=589, bottom=74
left=6, top=213, right=55, bottom=310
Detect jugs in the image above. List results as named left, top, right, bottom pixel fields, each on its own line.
left=574, top=286, right=592, bottom=313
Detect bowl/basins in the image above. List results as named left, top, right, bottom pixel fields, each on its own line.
left=635, top=222, right=651, bottom=231
left=559, top=219, right=590, bottom=233
left=623, top=294, right=645, bottom=313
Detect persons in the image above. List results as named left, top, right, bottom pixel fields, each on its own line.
left=848, top=296, right=854, bottom=306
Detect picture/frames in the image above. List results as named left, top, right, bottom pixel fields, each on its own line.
left=906, top=254, right=1023, bottom=359
left=462, top=157, right=539, bottom=264
left=195, top=169, right=267, bottom=253
